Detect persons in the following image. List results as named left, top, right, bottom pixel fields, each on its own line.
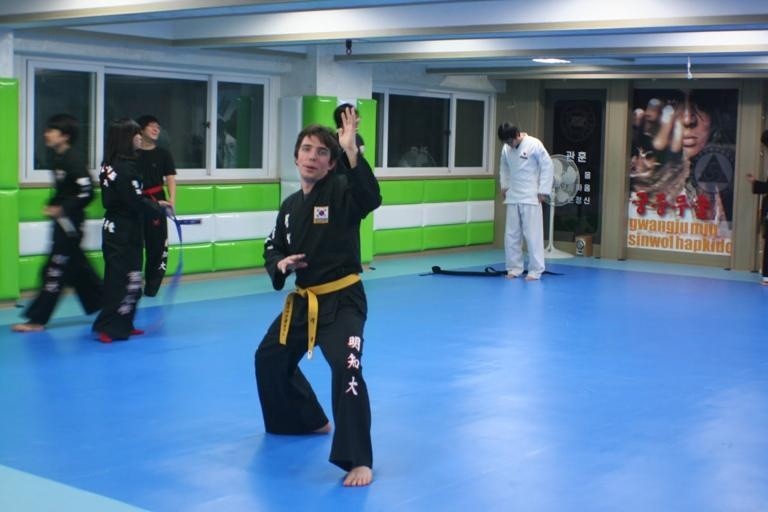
left=91, top=118, right=171, bottom=345
left=331, top=104, right=364, bottom=162
left=251, top=104, right=384, bottom=487
left=10, top=113, right=105, bottom=333
left=627, top=86, right=727, bottom=227
left=496, top=121, right=555, bottom=283
left=136, top=113, right=178, bottom=298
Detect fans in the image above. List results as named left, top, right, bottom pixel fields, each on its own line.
left=541, top=154, right=581, bottom=260
